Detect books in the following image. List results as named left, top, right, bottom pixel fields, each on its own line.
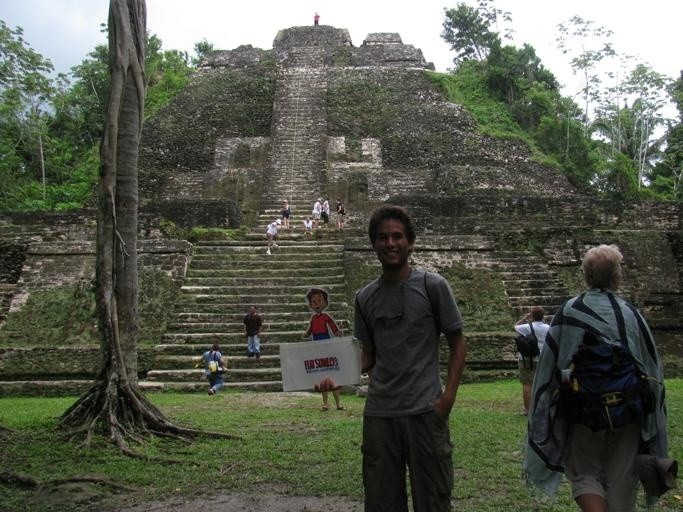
left=277, top=336, right=361, bottom=391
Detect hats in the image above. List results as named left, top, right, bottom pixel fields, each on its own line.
left=275, top=218, right=282, bottom=225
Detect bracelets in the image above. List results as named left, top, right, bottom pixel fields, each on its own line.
left=522, top=318, right=525, bottom=323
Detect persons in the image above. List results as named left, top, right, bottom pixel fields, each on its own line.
left=263, top=194, right=345, bottom=255
left=512, top=306, right=550, bottom=415
left=198, top=345, right=227, bottom=396
left=243, top=305, right=262, bottom=357
left=352, top=201, right=466, bottom=511
left=525, top=245, right=677, bottom=512
left=320, top=390, right=342, bottom=411
left=313, top=13, right=319, bottom=26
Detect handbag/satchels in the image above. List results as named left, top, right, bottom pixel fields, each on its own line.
left=207, top=361, right=228, bottom=375
left=514, top=334, right=540, bottom=359
left=556, top=343, right=649, bottom=431
